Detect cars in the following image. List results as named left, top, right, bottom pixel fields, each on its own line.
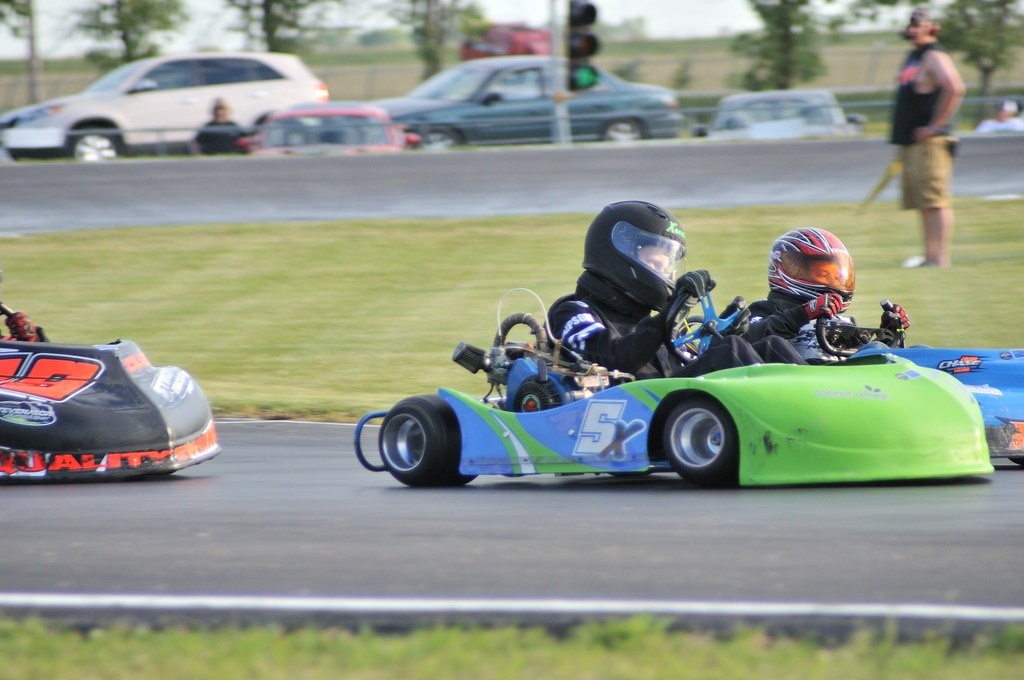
left=362, top=24, right=686, bottom=150
left=235, top=103, right=421, bottom=153
left=693, top=87, right=869, bottom=140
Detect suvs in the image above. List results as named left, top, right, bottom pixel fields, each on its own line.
left=0, top=50, right=330, bottom=160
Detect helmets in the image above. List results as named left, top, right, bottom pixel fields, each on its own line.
left=767, top=227, right=856, bottom=313
left=582, top=200, right=686, bottom=313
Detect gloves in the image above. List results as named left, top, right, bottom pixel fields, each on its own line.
left=803, top=293, right=843, bottom=320
left=718, top=296, right=751, bottom=337
left=880, top=304, right=910, bottom=336
left=661, top=270, right=716, bottom=323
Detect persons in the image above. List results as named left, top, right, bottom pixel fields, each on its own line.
left=194, top=103, right=249, bottom=154
left=734, top=228, right=908, bottom=365
left=976, top=97, right=1024, bottom=132
left=542, top=201, right=808, bottom=380
left=889, top=6, right=966, bottom=269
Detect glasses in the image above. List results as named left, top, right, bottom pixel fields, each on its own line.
left=911, top=17, right=930, bottom=27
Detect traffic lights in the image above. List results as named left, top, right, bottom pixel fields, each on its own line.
left=567, top=1, right=600, bottom=90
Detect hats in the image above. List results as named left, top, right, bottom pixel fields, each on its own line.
left=995, top=99, right=1018, bottom=112
